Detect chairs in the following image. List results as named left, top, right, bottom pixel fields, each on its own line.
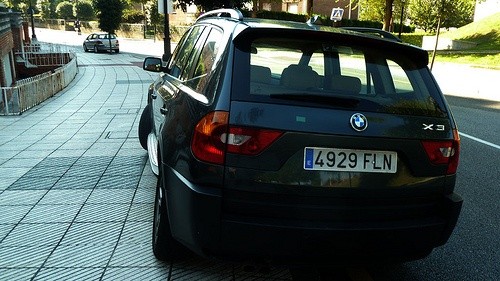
left=250, top=63, right=362, bottom=93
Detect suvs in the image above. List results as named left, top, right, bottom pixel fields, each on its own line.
left=136, top=8, right=465, bottom=262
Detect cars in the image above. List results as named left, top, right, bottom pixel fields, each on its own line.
left=83, top=32, right=120, bottom=54
